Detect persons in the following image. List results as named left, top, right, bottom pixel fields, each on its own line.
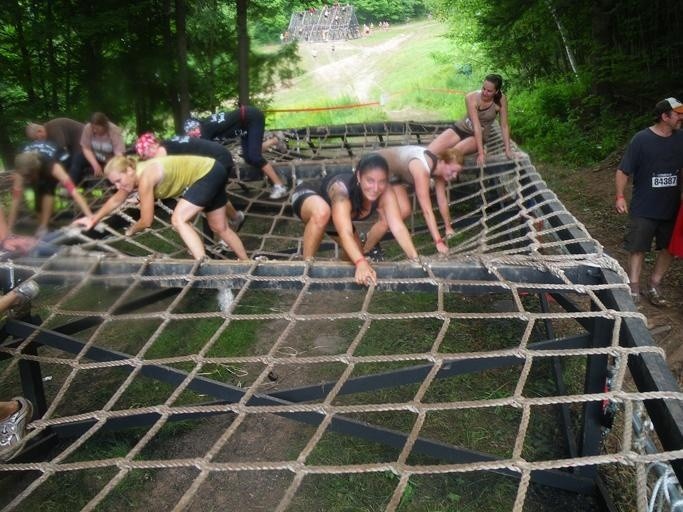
left=1, top=101, right=125, bottom=261
left=137, top=133, right=244, bottom=251
left=369, top=142, right=466, bottom=254
left=425, top=72, right=516, bottom=168
left=614, top=97, right=682, bottom=308
left=185, top=105, right=290, bottom=199
left=72, top=152, right=251, bottom=262
left=289, top=153, right=420, bottom=289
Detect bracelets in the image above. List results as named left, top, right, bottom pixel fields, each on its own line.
left=615, top=194, right=624, bottom=200
left=351, top=258, right=368, bottom=266
left=434, top=239, right=444, bottom=246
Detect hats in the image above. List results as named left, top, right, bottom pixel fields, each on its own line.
left=653, top=97, right=683, bottom=116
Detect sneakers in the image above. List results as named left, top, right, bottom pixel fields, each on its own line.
left=8, top=279, right=41, bottom=320
left=229, top=210, right=245, bottom=231
left=273, top=131, right=287, bottom=153
left=0, top=396, right=33, bottom=462
left=212, top=239, right=233, bottom=253
left=641, top=284, right=667, bottom=307
left=270, top=183, right=288, bottom=199
